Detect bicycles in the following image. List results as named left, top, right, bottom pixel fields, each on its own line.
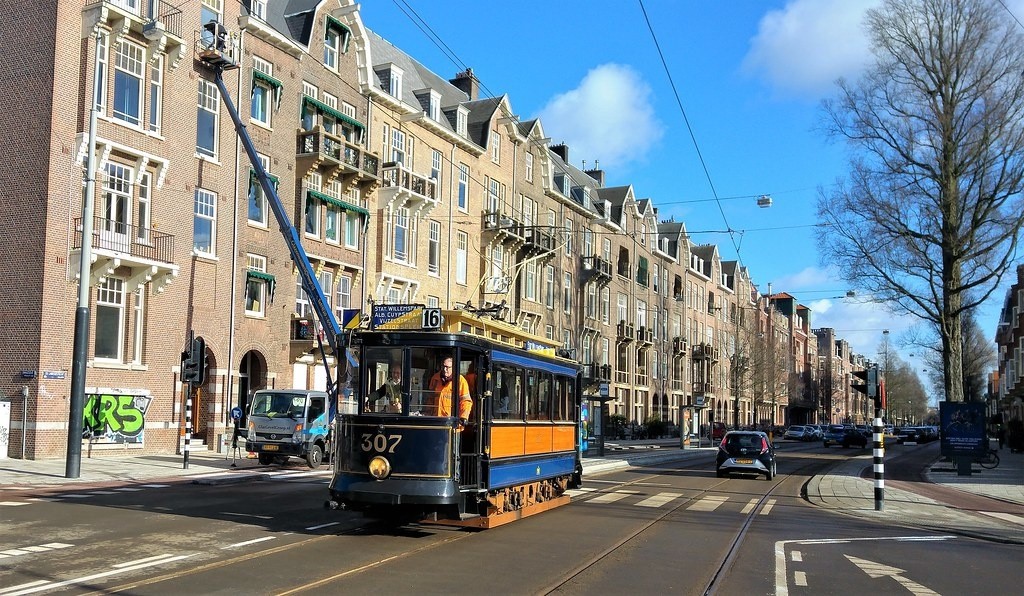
left=951, top=448, right=1000, bottom=469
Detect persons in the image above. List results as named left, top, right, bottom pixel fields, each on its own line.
left=425, top=357, right=472, bottom=482
left=365, top=362, right=403, bottom=413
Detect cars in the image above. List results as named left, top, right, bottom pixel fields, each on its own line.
left=884, top=424, right=895, bottom=435
left=707, top=423, right=727, bottom=441
left=805, top=423, right=875, bottom=441
left=783, top=425, right=809, bottom=441
left=823, top=426, right=867, bottom=449
left=894, top=425, right=941, bottom=444
left=715, top=431, right=779, bottom=481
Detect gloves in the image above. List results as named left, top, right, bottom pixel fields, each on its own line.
left=456, top=423, right=465, bottom=433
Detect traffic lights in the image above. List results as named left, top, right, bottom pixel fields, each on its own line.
left=182, top=361, right=197, bottom=383
left=849, top=369, right=876, bottom=399
left=196, top=337, right=209, bottom=390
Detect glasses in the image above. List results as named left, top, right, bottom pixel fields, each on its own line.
left=442, top=366, right=452, bottom=369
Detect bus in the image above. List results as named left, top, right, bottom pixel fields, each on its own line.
left=328, top=226, right=586, bottom=532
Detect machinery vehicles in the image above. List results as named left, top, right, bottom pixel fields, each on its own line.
left=199, top=28, right=363, bottom=470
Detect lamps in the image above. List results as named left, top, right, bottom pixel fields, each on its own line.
left=923, top=369, right=927, bottom=372
left=757, top=196, right=772, bottom=208
left=847, top=290, right=855, bottom=297
left=142, top=19, right=165, bottom=41
left=909, top=353, right=915, bottom=358
left=883, top=329, right=890, bottom=335
